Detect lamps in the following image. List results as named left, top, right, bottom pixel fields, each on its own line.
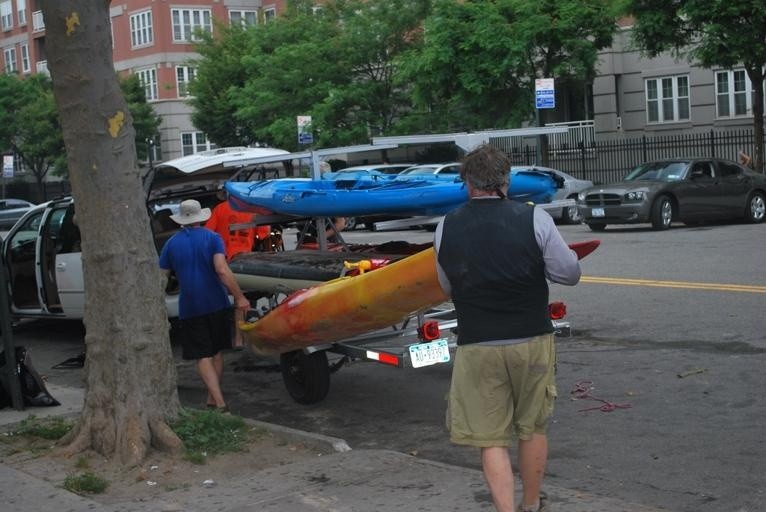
left=151, top=208, right=180, bottom=252
left=56, top=223, right=82, bottom=253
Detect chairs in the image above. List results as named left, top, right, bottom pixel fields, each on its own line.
left=518, top=490, right=548, bottom=512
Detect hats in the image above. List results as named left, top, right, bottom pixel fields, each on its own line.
left=577, top=157, right=764, bottom=229
left=328, top=162, right=596, bottom=232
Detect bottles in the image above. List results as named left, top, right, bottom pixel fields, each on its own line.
left=169, top=199, right=212, bottom=225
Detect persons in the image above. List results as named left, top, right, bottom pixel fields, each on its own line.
left=295, top=163, right=346, bottom=244
left=738, top=150, right=757, bottom=172
left=204, top=199, right=272, bottom=352
left=432, top=144, right=583, bottom=510
left=159, top=198, right=252, bottom=418
